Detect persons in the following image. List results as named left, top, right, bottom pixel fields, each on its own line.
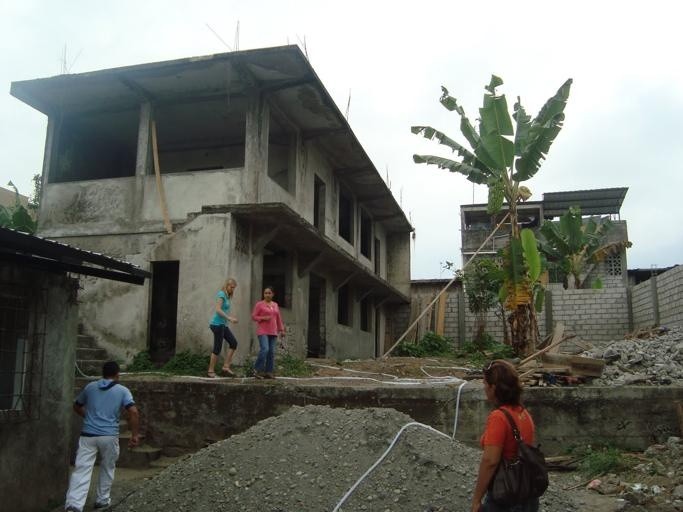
left=63, top=360, right=139, bottom=512
left=468, top=358, right=540, bottom=512
left=207, top=278, right=238, bottom=377
left=250, top=286, right=286, bottom=380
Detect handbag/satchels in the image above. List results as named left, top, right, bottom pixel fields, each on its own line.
left=488, top=442, right=548, bottom=507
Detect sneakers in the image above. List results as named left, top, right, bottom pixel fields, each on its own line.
left=94, top=498, right=112, bottom=512
left=253, top=368, right=275, bottom=379
left=222, top=367, right=236, bottom=377
left=207, top=372, right=220, bottom=378
